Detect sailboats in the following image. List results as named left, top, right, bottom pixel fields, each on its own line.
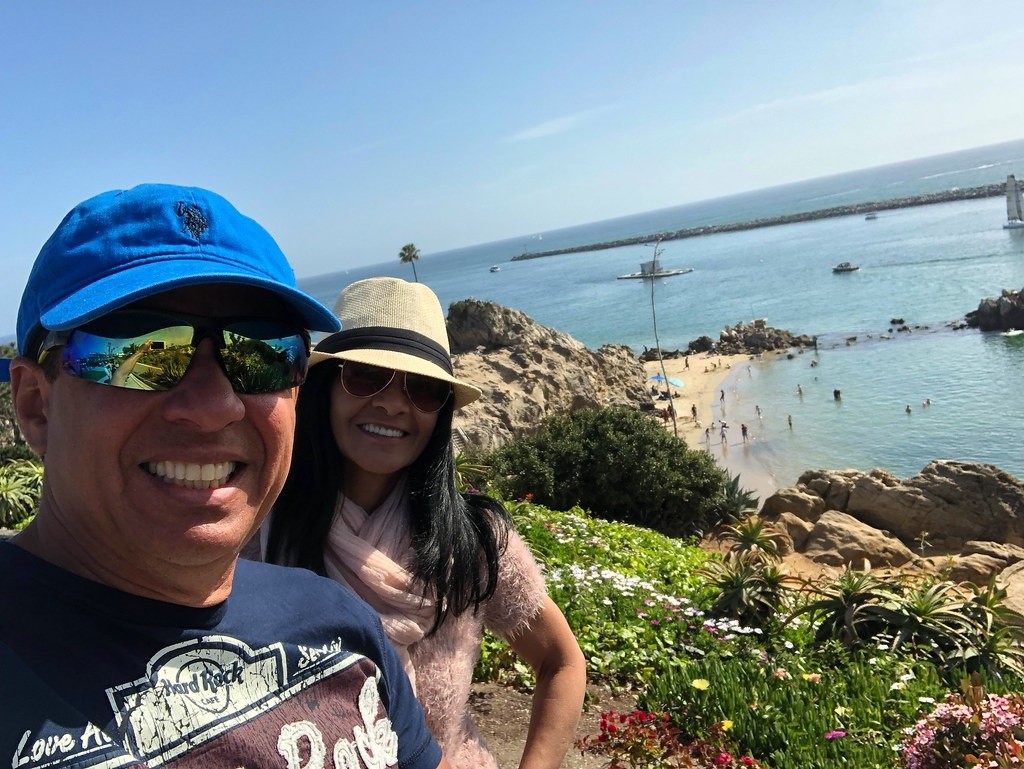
left=1002, top=174, right=1024, bottom=229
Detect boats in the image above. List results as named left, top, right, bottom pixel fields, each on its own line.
left=831, top=261, right=859, bottom=272
left=490, top=267, right=500, bottom=272
left=865, top=212, right=878, bottom=220
left=617, top=260, right=695, bottom=280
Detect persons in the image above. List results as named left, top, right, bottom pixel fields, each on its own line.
left=704, top=358, right=752, bottom=375
left=237, top=274, right=587, bottom=768
left=682, top=356, right=690, bottom=370
left=708, top=341, right=720, bottom=357
left=109, top=340, right=154, bottom=388
left=0, top=181, right=453, bottom=769
left=650, top=384, right=805, bottom=445
left=904, top=396, right=935, bottom=415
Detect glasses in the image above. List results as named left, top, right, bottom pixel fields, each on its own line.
left=35, top=308, right=311, bottom=394
left=337, top=360, right=454, bottom=413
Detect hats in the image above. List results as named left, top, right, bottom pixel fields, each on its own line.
left=15, top=184, right=342, bottom=356
left=310, top=277, right=482, bottom=410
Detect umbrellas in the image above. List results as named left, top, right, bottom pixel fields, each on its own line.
left=649, top=373, right=665, bottom=384
left=667, top=377, right=685, bottom=388
